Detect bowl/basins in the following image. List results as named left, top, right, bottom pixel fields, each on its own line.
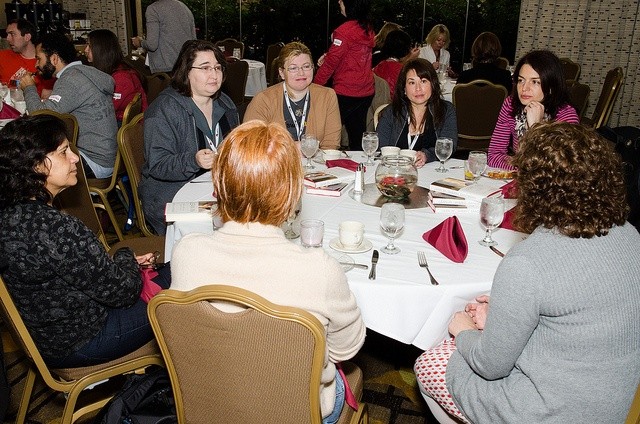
left=323, top=149, right=341, bottom=162
left=400, top=149, right=418, bottom=161
left=381, top=145, right=400, bottom=157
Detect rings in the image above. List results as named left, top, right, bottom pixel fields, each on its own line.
left=420, top=159, right=423, bottom=162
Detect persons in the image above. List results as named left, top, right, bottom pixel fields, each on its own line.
left=133, top=0, right=198, bottom=77
left=169, top=118, right=367, bottom=424
left=373, top=29, right=411, bottom=100
left=413, top=121, right=639, bottom=424
left=136, top=39, right=241, bottom=236
left=243, top=42, right=342, bottom=158
left=85, top=29, right=149, bottom=131
left=313, top=0, right=377, bottom=151
left=0, top=18, right=57, bottom=98
left=17, top=33, right=118, bottom=179
left=456, top=31, right=513, bottom=97
left=374, top=58, right=458, bottom=170
left=0, top=114, right=171, bottom=369
left=417, top=24, right=450, bottom=77
left=487, top=49, right=580, bottom=171
left=372, top=22, right=420, bottom=70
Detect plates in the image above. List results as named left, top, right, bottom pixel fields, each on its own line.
left=331, top=252, right=355, bottom=273
left=329, top=236, right=373, bottom=253
left=480, top=168, right=515, bottom=180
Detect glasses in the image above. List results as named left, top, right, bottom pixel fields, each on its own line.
left=139, top=251, right=160, bottom=266
left=193, top=66, right=225, bottom=73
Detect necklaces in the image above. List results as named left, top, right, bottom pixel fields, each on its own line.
left=288, top=93, right=307, bottom=117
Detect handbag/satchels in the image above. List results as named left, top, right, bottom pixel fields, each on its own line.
left=100, top=365, right=178, bottom=424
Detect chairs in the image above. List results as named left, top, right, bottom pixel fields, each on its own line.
left=117, top=113, right=158, bottom=237
left=27, top=109, right=165, bottom=264
left=215, top=38, right=245, bottom=59
left=581, top=66, right=624, bottom=129
left=452, top=79, right=508, bottom=158
left=217, top=60, right=249, bottom=124
left=89, top=92, right=141, bottom=240
left=0, top=277, right=166, bottom=424
left=146, top=284, right=369, bottom=424
left=557, top=57, right=581, bottom=87
left=496, top=56, right=509, bottom=71
left=265, top=41, right=286, bottom=86
left=147, top=73, right=170, bottom=103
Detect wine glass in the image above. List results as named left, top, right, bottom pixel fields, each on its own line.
left=379, top=202, right=406, bottom=255
left=0, top=83, right=9, bottom=103
left=434, top=137, right=453, bottom=173
left=467, top=150, right=488, bottom=187
left=300, top=134, right=318, bottom=170
left=479, top=196, right=505, bottom=247
left=362, top=131, right=379, bottom=166
left=284, top=198, right=302, bottom=239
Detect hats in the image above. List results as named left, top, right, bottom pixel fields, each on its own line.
left=282, top=65, right=314, bottom=73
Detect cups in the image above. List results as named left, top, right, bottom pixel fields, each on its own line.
left=13, top=100, right=27, bottom=115
left=233, top=48, right=241, bottom=60
left=299, top=218, right=325, bottom=248
left=339, top=221, right=363, bottom=248
left=464, top=160, right=475, bottom=180
left=10, top=87, right=25, bottom=104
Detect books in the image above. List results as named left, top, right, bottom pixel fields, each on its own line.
left=430, top=176, right=518, bottom=203
left=303, top=166, right=356, bottom=188
left=426, top=200, right=480, bottom=214
left=428, top=190, right=508, bottom=205
left=305, top=180, right=355, bottom=197
left=165, top=200, right=217, bottom=222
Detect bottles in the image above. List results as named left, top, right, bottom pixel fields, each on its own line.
left=354, top=166, right=363, bottom=193
left=359, top=162, right=365, bottom=187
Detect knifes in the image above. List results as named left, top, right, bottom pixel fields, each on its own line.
left=490, top=245, right=505, bottom=257
left=368, top=249, right=379, bottom=281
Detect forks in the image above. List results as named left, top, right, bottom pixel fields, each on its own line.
left=417, top=250, right=440, bottom=285
